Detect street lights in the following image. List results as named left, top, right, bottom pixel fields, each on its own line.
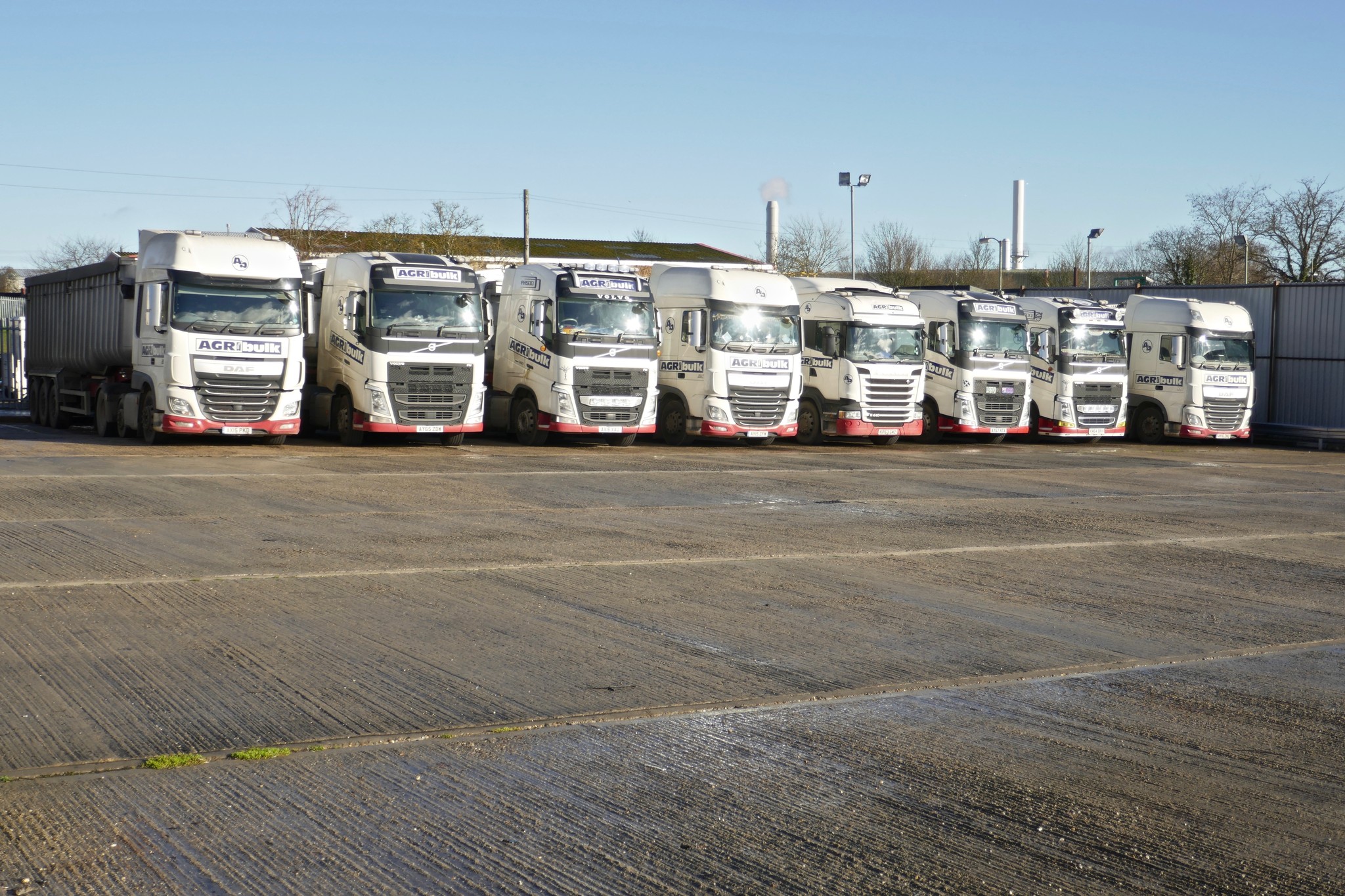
left=1085, top=228, right=1104, bottom=294
left=1235, top=236, right=1249, bottom=284
left=839, top=168, right=872, bottom=281
left=978, top=237, right=1005, bottom=293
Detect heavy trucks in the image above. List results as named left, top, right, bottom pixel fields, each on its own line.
left=1007, top=295, right=1130, bottom=443
left=25, top=233, right=319, bottom=448
left=474, top=262, right=664, bottom=448
left=893, top=290, right=1032, bottom=442
left=1103, top=293, right=1256, bottom=446
left=786, top=278, right=927, bottom=444
left=300, top=250, right=498, bottom=445
left=642, top=260, right=808, bottom=454
